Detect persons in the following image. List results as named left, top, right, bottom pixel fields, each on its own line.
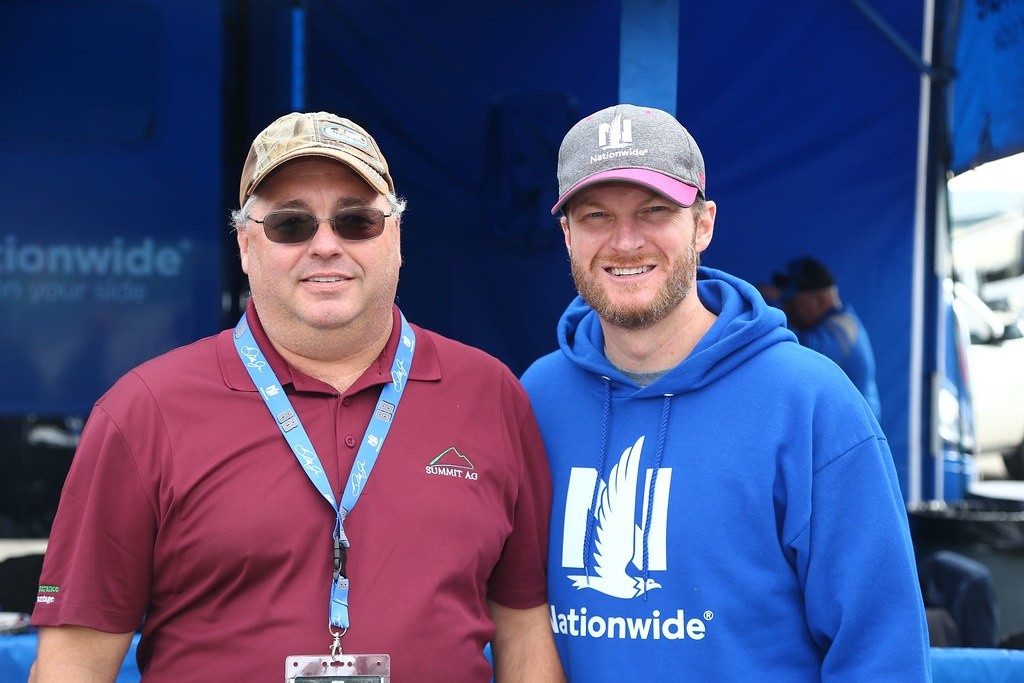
left=755, top=254, right=885, bottom=426
left=515, top=101, right=932, bottom=683
left=28, top=111, right=567, bottom=683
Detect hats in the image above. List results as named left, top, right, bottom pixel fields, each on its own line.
left=551, top=103, right=706, bottom=216
left=773, top=256, right=833, bottom=292
left=240, top=111, right=396, bottom=211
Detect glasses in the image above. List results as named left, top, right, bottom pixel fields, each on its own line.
left=247, top=207, right=391, bottom=244
